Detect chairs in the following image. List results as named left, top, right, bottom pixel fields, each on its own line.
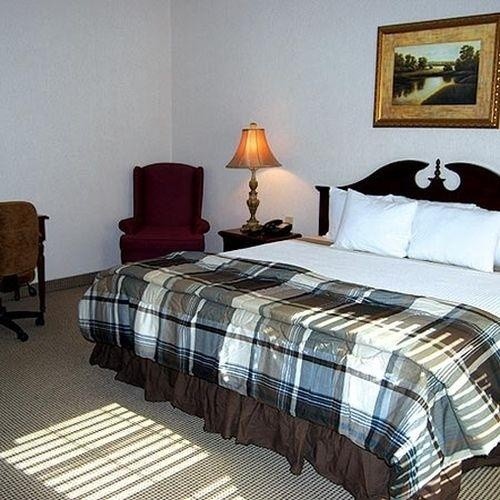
left=0, top=201, right=49, bottom=342
left=118, top=162, right=211, bottom=264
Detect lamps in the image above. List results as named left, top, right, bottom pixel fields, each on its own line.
left=225, top=122, right=282, bottom=233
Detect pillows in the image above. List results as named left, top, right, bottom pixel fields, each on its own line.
left=330, top=188, right=417, bottom=259
left=409, top=201, right=498, bottom=275
left=418, top=199, right=500, bottom=271
left=323, top=186, right=407, bottom=240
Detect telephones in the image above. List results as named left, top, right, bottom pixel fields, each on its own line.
left=260, top=219, right=293, bottom=239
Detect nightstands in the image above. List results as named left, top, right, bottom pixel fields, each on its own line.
left=217, top=224, right=301, bottom=253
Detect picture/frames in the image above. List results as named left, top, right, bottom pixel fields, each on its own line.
left=372, top=12, right=498, bottom=129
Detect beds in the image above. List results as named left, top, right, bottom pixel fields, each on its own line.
left=76, top=158, right=499, bottom=499
